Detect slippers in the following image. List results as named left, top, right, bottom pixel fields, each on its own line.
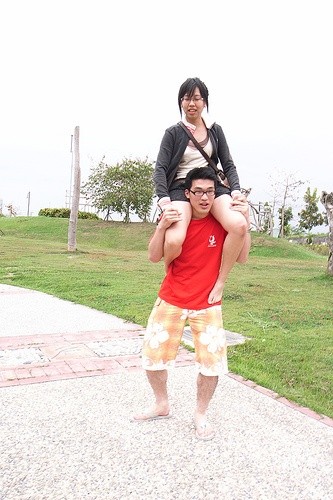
left=193, top=412, right=215, bottom=441
left=129, top=410, right=173, bottom=423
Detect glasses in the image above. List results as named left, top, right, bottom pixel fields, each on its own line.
left=181, top=97, right=204, bottom=103
left=188, top=188, right=216, bottom=196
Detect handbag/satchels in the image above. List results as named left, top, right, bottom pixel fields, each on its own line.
left=216, top=169, right=230, bottom=189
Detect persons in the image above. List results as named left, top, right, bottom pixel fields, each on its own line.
left=131, top=166, right=250, bottom=442
left=153, top=77, right=247, bottom=304
